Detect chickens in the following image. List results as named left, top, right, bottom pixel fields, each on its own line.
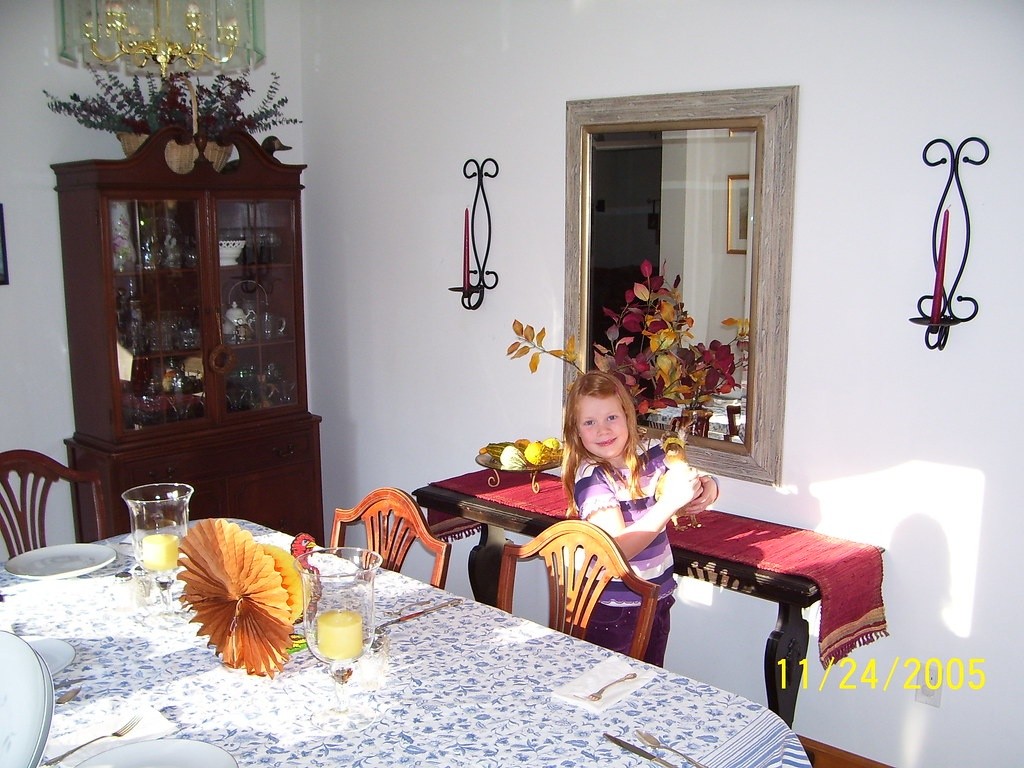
left=654, top=432, right=720, bottom=531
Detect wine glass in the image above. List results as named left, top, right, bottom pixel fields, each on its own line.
left=293, top=548, right=377, bottom=732
left=114, top=289, right=299, bottom=427
left=121, top=480, right=194, bottom=632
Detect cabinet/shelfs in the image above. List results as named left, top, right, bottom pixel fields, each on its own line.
left=50, top=128, right=325, bottom=549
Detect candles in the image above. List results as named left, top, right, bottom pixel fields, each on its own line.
left=931, top=209, right=949, bottom=324
left=317, top=610, right=362, bottom=658
left=143, top=534, right=178, bottom=571
left=463, top=208, right=469, bottom=290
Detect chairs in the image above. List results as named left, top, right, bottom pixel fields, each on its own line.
left=727, top=405, right=741, bottom=436
left=0, top=450, right=660, bottom=658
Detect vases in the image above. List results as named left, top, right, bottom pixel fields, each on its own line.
left=683, top=408, right=713, bottom=438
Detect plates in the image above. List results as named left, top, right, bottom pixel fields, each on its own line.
left=4, top=542, right=117, bottom=580
left=1, top=628, right=53, bottom=768
left=27, top=636, right=75, bottom=678
left=73, top=738, right=240, bottom=768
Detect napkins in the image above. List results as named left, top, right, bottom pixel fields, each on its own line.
left=106, top=529, right=148, bottom=558
left=552, top=654, right=658, bottom=713
left=43, top=705, right=178, bottom=768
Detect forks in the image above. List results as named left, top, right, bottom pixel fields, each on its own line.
left=573, top=672, right=639, bottom=702
left=43, top=716, right=146, bottom=767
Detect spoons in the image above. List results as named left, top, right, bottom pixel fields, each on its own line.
left=636, top=728, right=708, bottom=768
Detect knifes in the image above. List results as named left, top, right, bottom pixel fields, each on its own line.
left=377, top=598, right=463, bottom=629
left=603, top=734, right=678, bottom=768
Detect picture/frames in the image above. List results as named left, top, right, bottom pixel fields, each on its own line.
left=727, top=175, right=749, bottom=254
left=0, top=204, right=9, bottom=286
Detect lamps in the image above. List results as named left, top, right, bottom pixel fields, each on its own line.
left=58, top=0, right=266, bottom=79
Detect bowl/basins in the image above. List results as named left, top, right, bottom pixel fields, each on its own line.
left=218, top=239, right=245, bottom=267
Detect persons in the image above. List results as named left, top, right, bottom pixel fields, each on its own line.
left=564, top=371, right=721, bottom=670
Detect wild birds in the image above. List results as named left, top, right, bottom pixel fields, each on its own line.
left=176, top=517, right=325, bottom=680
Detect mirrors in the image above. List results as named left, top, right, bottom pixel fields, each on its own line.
left=563, top=85, right=799, bottom=488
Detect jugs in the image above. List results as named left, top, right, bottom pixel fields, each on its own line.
left=138, top=235, right=197, bottom=269
left=161, top=356, right=193, bottom=392
left=221, top=301, right=255, bottom=335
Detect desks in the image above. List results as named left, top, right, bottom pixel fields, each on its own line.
left=411, top=468, right=889, bottom=768
left=0, top=518, right=812, bottom=768
left=647, top=398, right=748, bottom=445
